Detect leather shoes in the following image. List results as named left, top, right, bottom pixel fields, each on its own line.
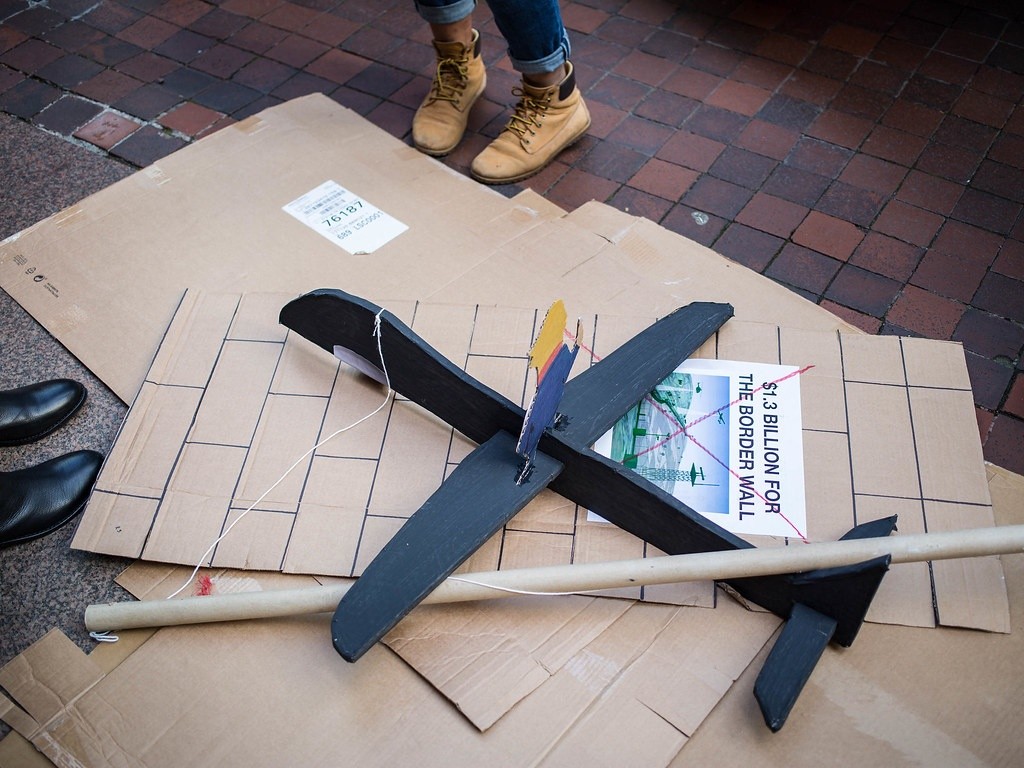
left=0, top=379, right=87, bottom=447
left=0, top=451, right=105, bottom=549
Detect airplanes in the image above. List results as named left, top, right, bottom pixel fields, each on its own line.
left=280, top=288, right=898, bottom=734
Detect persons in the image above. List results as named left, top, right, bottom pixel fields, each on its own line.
left=0, top=377, right=105, bottom=552
left=412, top=0, right=592, bottom=185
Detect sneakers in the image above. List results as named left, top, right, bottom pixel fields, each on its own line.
left=412, top=27, right=487, bottom=155
left=469, top=60, right=592, bottom=184
